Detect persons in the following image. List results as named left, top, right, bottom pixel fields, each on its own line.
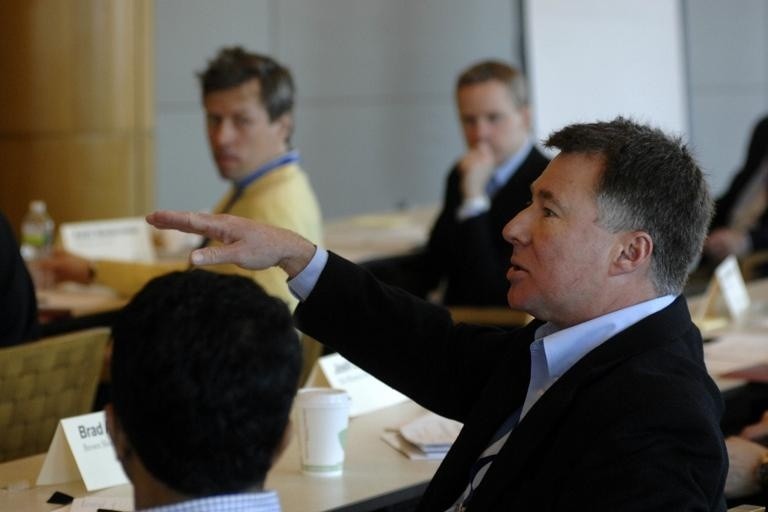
left=140, top=114, right=732, bottom=512
left=686, top=111, right=767, bottom=297
left=399, top=59, right=553, bottom=313
left=34, top=44, right=330, bottom=411
left=103, top=265, right=303, bottom=512
left=718, top=380, right=767, bottom=511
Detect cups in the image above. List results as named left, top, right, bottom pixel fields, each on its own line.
left=295, top=388, right=349, bottom=479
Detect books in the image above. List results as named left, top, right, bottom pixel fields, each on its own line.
left=381, top=413, right=463, bottom=461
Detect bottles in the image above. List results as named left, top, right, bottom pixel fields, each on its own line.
left=20, top=199, right=54, bottom=290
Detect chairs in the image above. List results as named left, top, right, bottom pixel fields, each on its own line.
left=0, top=326, right=110, bottom=463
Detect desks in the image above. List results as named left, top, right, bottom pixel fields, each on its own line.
left=29, top=201, right=441, bottom=323
left=1, top=263, right=768, bottom=510
